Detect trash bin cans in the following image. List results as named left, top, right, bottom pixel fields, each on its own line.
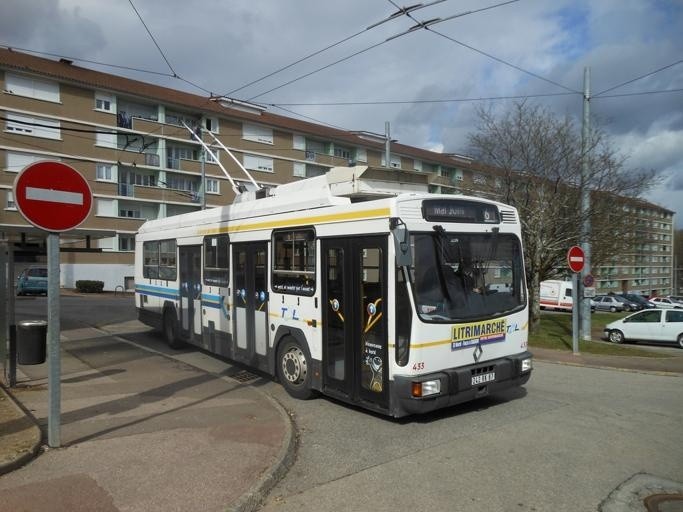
left=17, top=320, right=47, bottom=365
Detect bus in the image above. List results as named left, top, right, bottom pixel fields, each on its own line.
left=132, top=164, right=534, bottom=420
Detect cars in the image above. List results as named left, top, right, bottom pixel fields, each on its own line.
left=592, top=293, right=682, bottom=313
left=16, top=264, right=49, bottom=296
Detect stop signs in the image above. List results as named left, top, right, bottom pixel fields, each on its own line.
left=13, top=161, right=94, bottom=234
left=567, top=246, right=584, bottom=273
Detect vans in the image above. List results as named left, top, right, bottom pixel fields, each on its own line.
left=603, top=308, right=682, bottom=348
left=538, top=280, right=597, bottom=314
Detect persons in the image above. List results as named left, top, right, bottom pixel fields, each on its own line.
left=440, top=256, right=482, bottom=309
